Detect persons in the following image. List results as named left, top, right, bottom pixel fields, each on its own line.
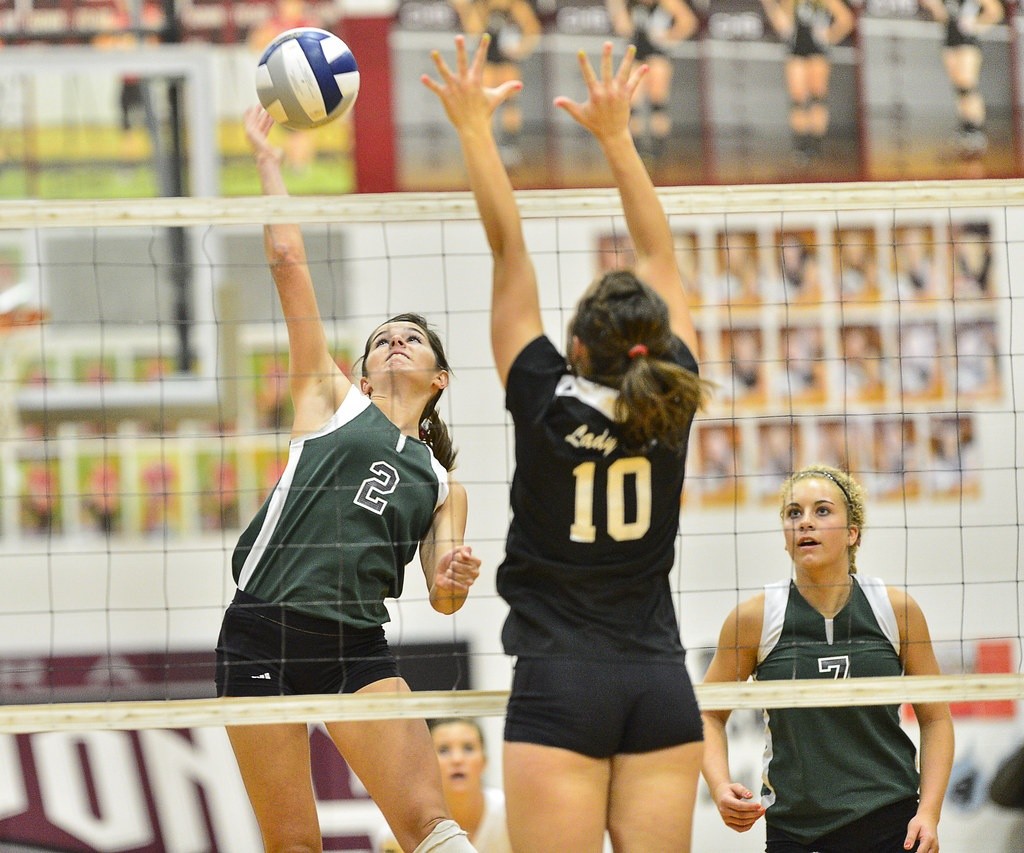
left=451, top=2, right=542, bottom=163
left=601, top=222, right=1002, bottom=492
left=697, top=466, right=955, bottom=853
left=761, top=0, right=855, bottom=165
left=249, top=0, right=321, bottom=170
left=923, top=0, right=1005, bottom=161
left=211, top=103, right=483, bottom=853
left=609, top=1, right=699, bottom=158
left=378, top=718, right=512, bottom=853
left=420, top=33, right=703, bottom=853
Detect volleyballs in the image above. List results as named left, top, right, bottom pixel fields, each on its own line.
left=255, top=24, right=364, bottom=130
